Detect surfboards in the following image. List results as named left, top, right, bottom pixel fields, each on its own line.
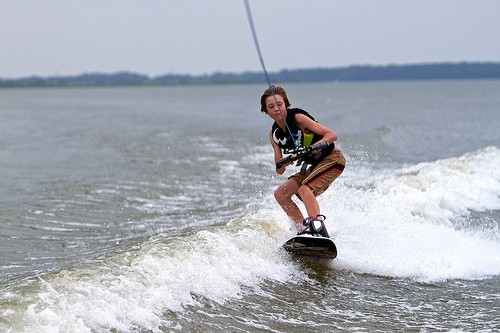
left=282, top=236, right=337, bottom=259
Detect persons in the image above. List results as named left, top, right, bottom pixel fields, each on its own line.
left=260, top=84, right=347, bottom=238
